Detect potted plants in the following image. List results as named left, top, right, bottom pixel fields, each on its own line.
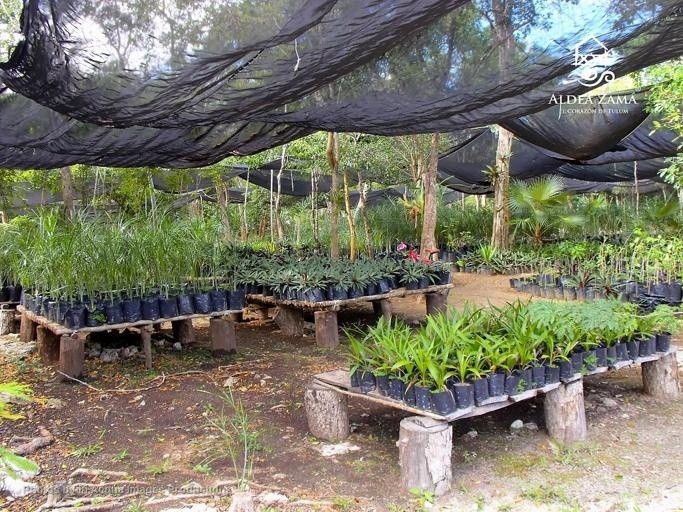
left=0, top=197, right=683, bottom=415
